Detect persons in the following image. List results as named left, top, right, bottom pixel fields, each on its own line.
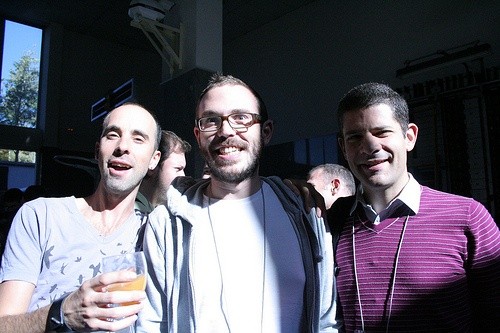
left=135, top=72, right=339, bottom=333
left=134, top=129, right=192, bottom=226
left=283, top=83, right=500, bottom=333
left=0, top=102, right=161, bottom=333
left=305, top=163, right=355, bottom=210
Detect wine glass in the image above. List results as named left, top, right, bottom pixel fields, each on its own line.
left=101, top=251, right=147, bottom=333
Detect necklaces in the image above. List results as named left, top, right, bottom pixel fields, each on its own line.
left=353, top=209, right=406, bottom=234
left=350, top=210, right=410, bottom=333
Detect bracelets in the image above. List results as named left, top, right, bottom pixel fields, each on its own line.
left=45, top=291, right=73, bottom=333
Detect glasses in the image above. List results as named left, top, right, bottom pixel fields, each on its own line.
left=195, top=112, right=263, bottom=133
